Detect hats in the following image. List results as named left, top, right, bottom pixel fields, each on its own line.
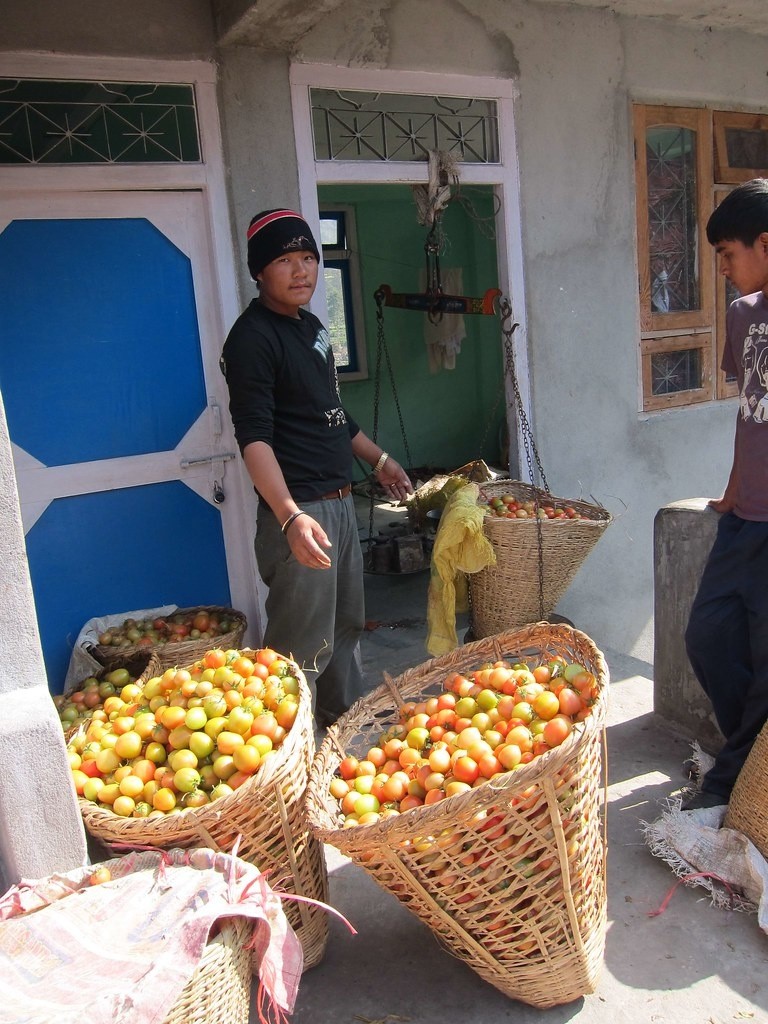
left=246, top=209, right=320, bottom=279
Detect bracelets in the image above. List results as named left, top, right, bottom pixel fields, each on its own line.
left=371, top=452, right=388, bottom=476
left=282, top=511, right=303, bottom=535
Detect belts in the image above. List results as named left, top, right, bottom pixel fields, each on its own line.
left=297, top=484, right=351, bottom=501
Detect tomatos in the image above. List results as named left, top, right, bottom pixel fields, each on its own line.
left=49, top=609, right=327, bottom=935
left=477, top=494, right=591, bottom=521
left=328, top=655, right=603, bottom=963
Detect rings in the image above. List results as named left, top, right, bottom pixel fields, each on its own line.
left=391, top=483, right=395, bottom=486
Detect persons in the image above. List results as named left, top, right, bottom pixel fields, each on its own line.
left=684, top=178, right=767, bottom=811
left=221, top=208, right=413, bottom=725
